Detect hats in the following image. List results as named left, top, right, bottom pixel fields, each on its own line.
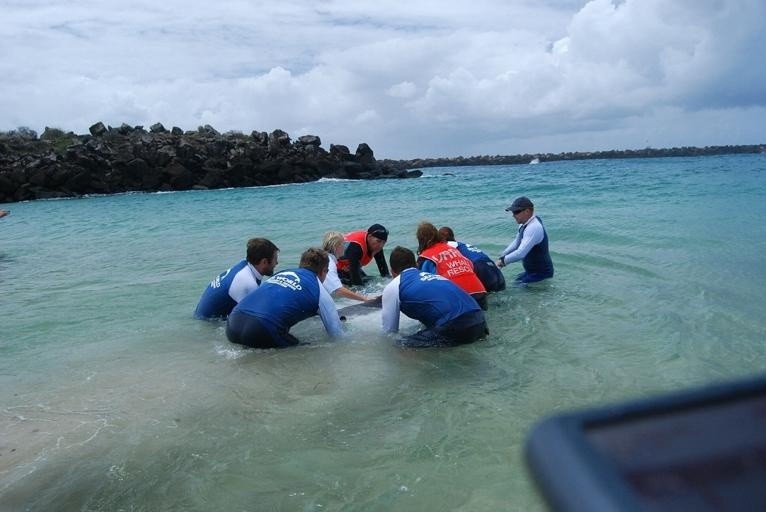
left=506, top=197, right=533, bottom=212
left=368, top=223, right=389, bottom=240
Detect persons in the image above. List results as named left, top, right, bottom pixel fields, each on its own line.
left=381, top=222, right=507, bottom=349
left=195, top=237, right=281, bottom=319
left=495, top=196, right=552, bottom=283
left=225, top=247, right=346, bottom=348
left=320, top=224, right=393, bottom=303
